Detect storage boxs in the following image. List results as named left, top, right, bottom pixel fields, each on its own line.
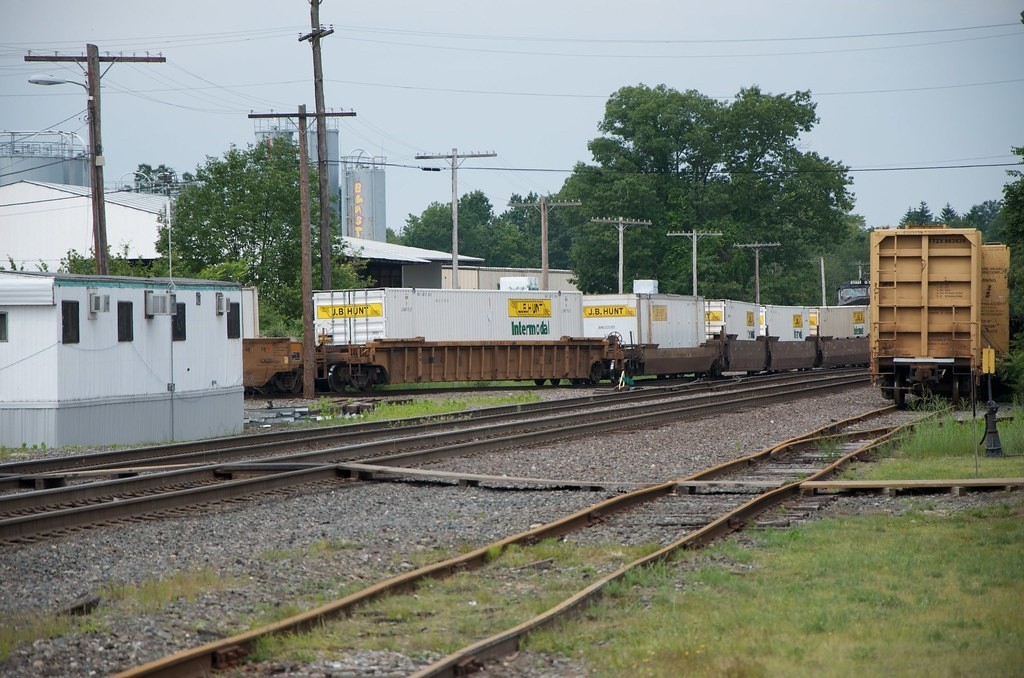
left=313, top=231, right=1009, bottom=367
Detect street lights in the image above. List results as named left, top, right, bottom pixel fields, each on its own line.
left=29, top=75, right=109, bottom=274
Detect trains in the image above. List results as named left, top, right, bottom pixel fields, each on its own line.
left=240, top=332, right=869, bottom=393
left=863, top=227, right=1013, bottom=412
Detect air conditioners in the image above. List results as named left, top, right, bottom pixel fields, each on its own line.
left=145, top=293, right=169, bottom=315
left=218, top=297, right=230, bottom=313
left=90, top=295, right=101, bottom=312
